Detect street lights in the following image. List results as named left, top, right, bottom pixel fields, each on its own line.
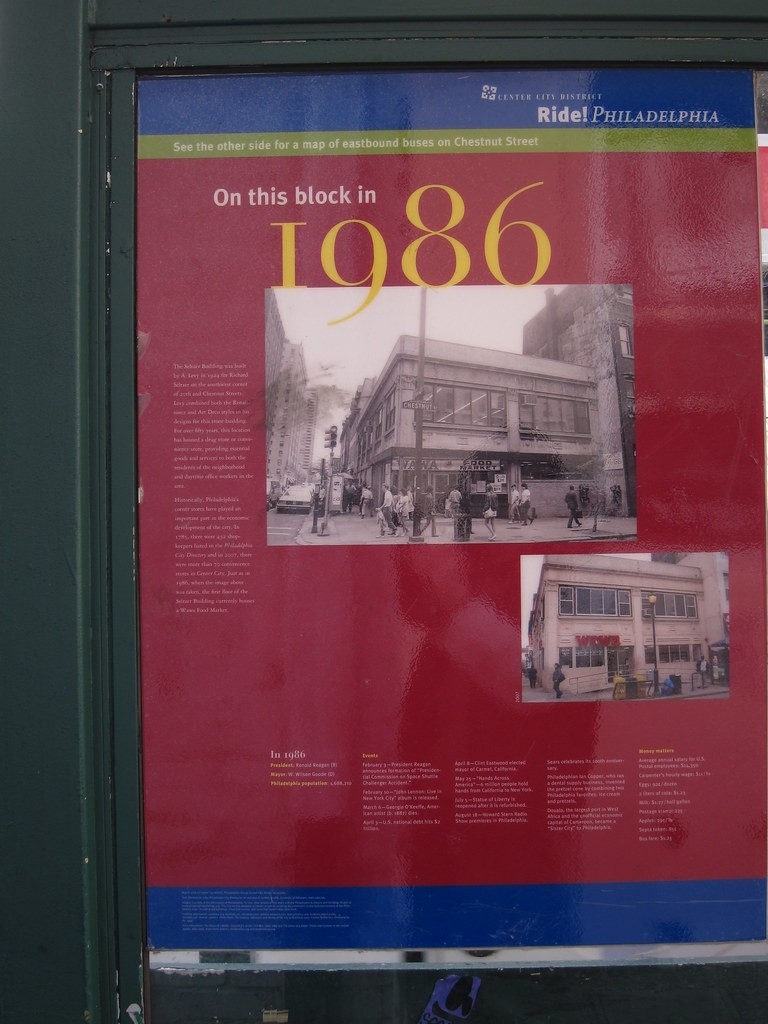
left=648, top=594, right=661, bottom=698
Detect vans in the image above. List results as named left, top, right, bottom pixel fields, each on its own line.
left=266, top=480, right=281, bottom=509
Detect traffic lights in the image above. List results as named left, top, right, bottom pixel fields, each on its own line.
left=324, top=425, right=337, bottom=449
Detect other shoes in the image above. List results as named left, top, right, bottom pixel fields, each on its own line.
left=578, top=523, right=582, bottom=526
left=521, top=523, right=527, bottom=527
left=432, top=534, right=439, bottom=537
left=376, top=534, right=384, bottom=538
left=401, top=530, right=407, bottom=536
left=488, top=535, right=497, bottom=541
left=567, top=526, right=574, bottom=528
left=508, top=521, right=514, bottom=524
left=387, top=533, right=398, bottom=537
left=530, top=518, right=534, bottom=524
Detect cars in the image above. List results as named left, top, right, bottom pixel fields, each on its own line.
left=276, top=484, right=315, bottom=514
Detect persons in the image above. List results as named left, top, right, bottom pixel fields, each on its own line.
left=552, top=663, right=563, bottom=698
left=528, top=665, right=538, bottom=688
left=342, top=480, right=624, bottom=540
left=696, top=655, right=709, bottom=684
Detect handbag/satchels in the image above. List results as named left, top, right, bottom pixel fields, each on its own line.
left=574, top=510, right=583, bottom=518
left=557, top=672, right=565, bottom=682
left=482, top=508, right=493, bottom=519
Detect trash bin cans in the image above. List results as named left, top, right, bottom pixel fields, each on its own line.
left=453, top=511, right=471, bottom=542
left=612, top=674, right=647, bottom=700
left=669, top=673, right=682, bottom=694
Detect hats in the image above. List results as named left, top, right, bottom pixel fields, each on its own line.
left=382, top=482, right=389, bottom=487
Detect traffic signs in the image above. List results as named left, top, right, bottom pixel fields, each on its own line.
left=402, top=400, right=438, bottom=412
